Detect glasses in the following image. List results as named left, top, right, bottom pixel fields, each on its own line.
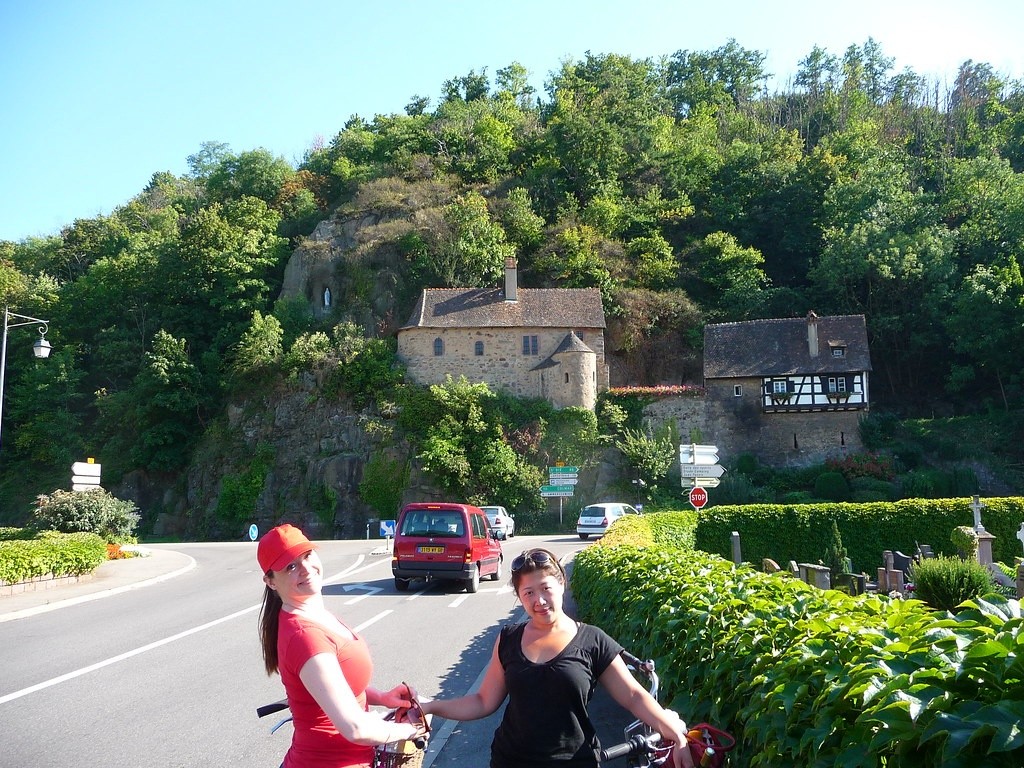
left=511, top=551, right=563, bottom=577
left=401, top=681, right=432, bottom=735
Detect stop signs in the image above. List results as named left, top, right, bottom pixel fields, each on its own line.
left=689, top=487, right=708, bottom=508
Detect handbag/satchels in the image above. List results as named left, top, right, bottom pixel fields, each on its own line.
left=654, top=722, right=735, bottom=768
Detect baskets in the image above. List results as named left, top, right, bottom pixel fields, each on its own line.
left=374, top=740, right=424, bottom=768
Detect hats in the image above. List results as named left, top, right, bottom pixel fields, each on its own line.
left=258, top=524, right=319, bottom=574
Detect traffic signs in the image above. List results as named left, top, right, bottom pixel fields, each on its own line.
left=71, top=475, right=100, bottom=484
left=680, top=453, right=720, bottom=464
left=549, top=467, right=579, bottom=473
left=72, top=483, right=99, bottom=492
left=71, top=461, right=101, bottom=477
left=540, top=492, right=573, bottom=497
left=550, top=479, right=579, bottom=485
left=549, top=474, right=579, bottom=479
left=539, top=486, right=574, bottom=492
left=681, top=476, right=721, bottom=488
left=679, top=444, right=719, bottom=454
left=681, top=465, right=727, bottom=478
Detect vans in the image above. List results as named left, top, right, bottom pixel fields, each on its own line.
left=391, top=502, right=504, bottom=593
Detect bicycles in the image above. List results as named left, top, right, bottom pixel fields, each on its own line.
left=256, top=698, right=429, bottom=768
left=601, top=650, right=730, bottom=768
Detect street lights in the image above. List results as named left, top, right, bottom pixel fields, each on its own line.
left=0, top=305, right=54, bottom=436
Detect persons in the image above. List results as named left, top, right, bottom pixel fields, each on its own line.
left=257, top=524, right=430, bottom=768
left=395, top=548, right=692, bottom=768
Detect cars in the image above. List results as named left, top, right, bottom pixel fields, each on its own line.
left=477, top=506, right=516, bottom=540
left=576, top=502, right=645, bottom=539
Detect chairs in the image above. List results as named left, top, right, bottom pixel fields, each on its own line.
left=414, top=522, right=428, bottom=531
left=431, top=521, right=448, bottom=530
